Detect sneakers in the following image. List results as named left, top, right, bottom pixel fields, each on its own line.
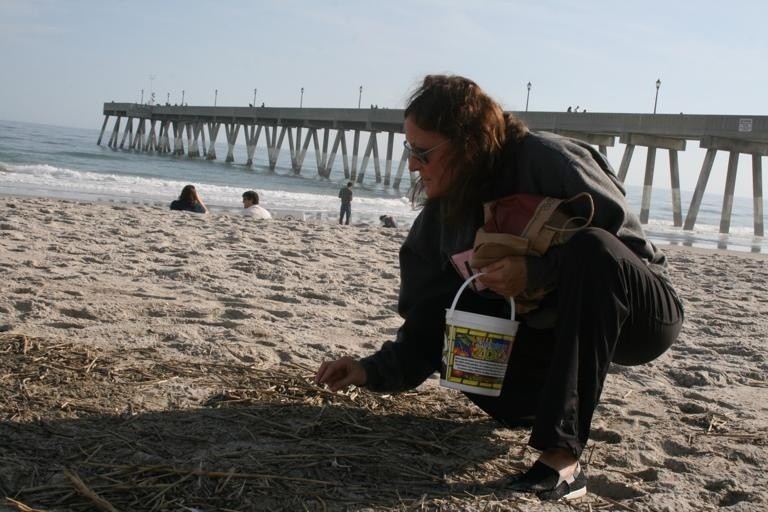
left=507, top=461, right=587, bottom=502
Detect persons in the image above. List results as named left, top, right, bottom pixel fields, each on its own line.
left=170, top=186, right=206, bottom=213
left=568, top=105, right=586, bottom=112
left=379, top=215, right=397, bottom=228
left=239, top=191, right=271, bottom=219
left=339, top=182, right=353, bottom=226
left=314, top=76, right=685, bottom=503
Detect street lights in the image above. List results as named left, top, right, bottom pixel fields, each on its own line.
left=654, top=79, right=661, bottom=113
left=300, top=88, right=304, bottom=108
left=142, top=90, right=184, bottom=106
left=253, top=89, right=256, bottom=108
left=358, top=85, right=362, bottom=108
left=215, top=89, right=217, bottom=106
left=525, top=82, right=531, bottom=112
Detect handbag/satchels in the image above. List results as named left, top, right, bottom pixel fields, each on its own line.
left=471, top=191, right=594, bottom=314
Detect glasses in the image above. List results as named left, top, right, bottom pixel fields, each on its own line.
left=404, top=139, right=451, bottom=164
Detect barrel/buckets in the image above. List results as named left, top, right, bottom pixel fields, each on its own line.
left=438, top=272, right=521, bottom=397
